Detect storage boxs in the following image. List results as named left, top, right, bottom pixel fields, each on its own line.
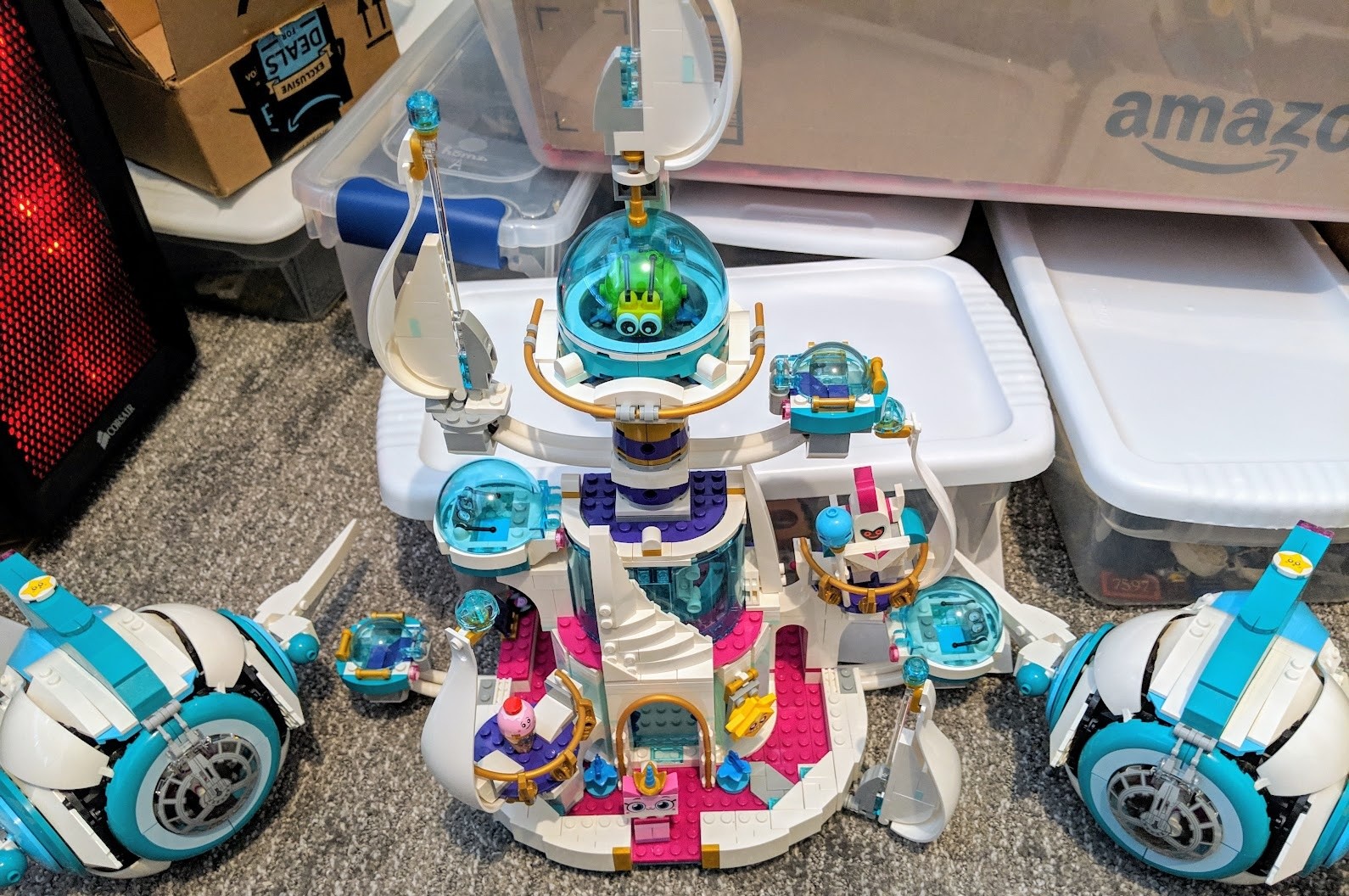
left=0, top=0, right=1349, bottom=599
